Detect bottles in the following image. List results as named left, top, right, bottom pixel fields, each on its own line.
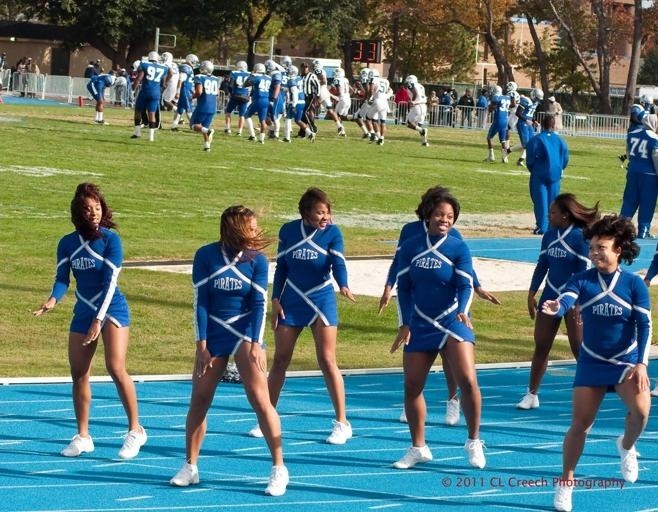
left=548, top=96, right=555, bottom=102
left=96, top=58, right=101, bottom=62
left=27, top=57, right=33, bottom=61
left=121, top=68, right=126, bottom=72
left=300, top=61, right=310, bottom=67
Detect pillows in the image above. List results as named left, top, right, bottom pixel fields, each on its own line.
left=507, top=80, right=518, bottom=92
left=236, top=61, right=248, bottom=72
left=115, top=77, right=127, bottom=88
left=264, top=58, right=271, bottom=65
left=490, top=85, right=503, bottom=95
left=253, top=63, right=266, bottom=75
left=360, top=67, right=370, bottom=83
left=199, top=60, right=217, bottom=77
left=334, top=68, right=345, bottom=79
left=639, top=93, right=655, bottom=109
left=268, top=61, right=277, bottom=70
left=161, top=52, right=172, bottom=63
left=280, top=56, right=292, bottom=69
left=147, top=50, right=161, bottom=64
left=530, top=88, right=544, bottom=103
left=368, top=69, right=380, bottom=80
left=288, top=65, right=298, bottom=76
left=184, top=54, right=200, bottom=69
left=131, top=59, right=142, bottom=71
left=404, top=75, right=418, bottom=85
left=313, top=60, right=323, bottom=70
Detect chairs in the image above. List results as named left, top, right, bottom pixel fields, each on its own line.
left=554, top=478, right=574, bottom=512
left=309, top=132, right=317, bottom=143
left=616, top=151, right=626, bottom=170
left=249, top=422, right=264, bottom=441
left=447, top=398, right=458, bottom=425
left=421, top=143, right=429, bottom=147
left=94, top=119, right=98, bottom=123
left=420, top=128, right=428, bottom=144
left=201, top=147, right=211, bottom=153
left=516, top=161, right=526, bottom=167
left=177, top=118, right=185, bottom=125
left=502, top=156, right=508, bottom=164
left=265, top=465, right=292, bottom=497
left=515, top=393, right=540, bottom=409
left=224, top=129, right=232, bottom=134
left=255, top=139, right=266, bottom=146
left=618, top=435, right=641, bottom=482
left=647, top=233, right=656, bottom=238
left=246, top=135, right=257, bottom=142
left=206, top=129, right=215, bottom=145
left=362, top=132, right=371, bottom=140
left=483, top=158, right=495, bottom=163
left=170, top=126, right=182, bottom=135
left=325, top=418, right=353, bottom=446
left=128, top=135, right=141, bottom=139
left=98, top=120, right=110, bottom=126
left=376, top=137, right=384, bottom=146
left=278, top=137, right=291, bottom=144
left=368, top=133, right=379, bottom=144
left=464, top=437, right=488, bottom=469
left=117, top=427, right=149, bottom=460
left=400, top=407, right=408, bottom=424
left=169, top=462, right=201, bottom=487
left=506, top=149, right=510, bottom=158
left=340, top=133, right=347, bottom=137
left=178, top=117, right=186, bottom=126
left=235, top=133, right=243, bottom=137
left=140, top=122, right=148, bottom=129
left=650, top=383, right=657, bottom=400
left=336, top=127, right=344, bottom=135
left=392, top=444, right=433, bottom=470
left=146, top=138, right=154, bottom=143
left=61, top=433, right=95, bottom=458
left=267, top=128, right=274, bottom=138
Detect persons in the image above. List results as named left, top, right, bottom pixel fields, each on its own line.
left=459, top=88, right=474, bottom=129
left=541, top=215, right=653, bottom=512
left=309, top=61, right=346, bottom=138
left=86, top=73, right=118, bottom=126
left=189, top=60, right=220, bottom=152
left=354, top=66, right=375, bottom=140
left=85, top=59, right=104, bottom=79
left=18, top=57, right=40, bottom=98
left=280, top=65, right=316, bottom=144
left=379, top=185, right=484, bottom=425
left=526, top=114, right=569, bottom=235
left=427, top=88, right=458, bottom=127
left=172, top=205, right=289, bottom=495
left=390, top=191, right=484, bottom=469
left=619, top=95, right=654, bottom=168
left=328, top=84, right=337, bottom=109
left=33, top=183, right=148, bottom=457
left=548, top=96, right=563, bottom=134
left=644, top=239, right=658, bottom=362
left=406, top=75, right=429, bottom=147
left=477, top=90, right=488, bottom=130
left=244, top=63, right=272, bottom=144
left=170, top=54, right=199, bottom=133
left=265, top=60, right=283, bottom=141
left=505, top=82, right=521, bottom=153
left=293, top=63, right=320, bottom=141
left=369, top=70, right=392, bottom=146
left=249, top=188, right=353, bottom=445
left=620, top=113, right=658, bottom=241
left=516, top=193, right=603, bottom=410
left=159, top=52, right=178, bottom=125
left=335, top=67, right=360, bottom=137
left=0, top=52, right=8, bottom=103
left=394, top=84, right=413, bottom=125
left=504, top=87, right=544, bottom=168
left=113, top=62, right=130, bottom=104
left=131, top=51, right=169, bottom=141
left=224, top=61, right=251, bottom=136
left=482, top=85, right=508, bottom=164
